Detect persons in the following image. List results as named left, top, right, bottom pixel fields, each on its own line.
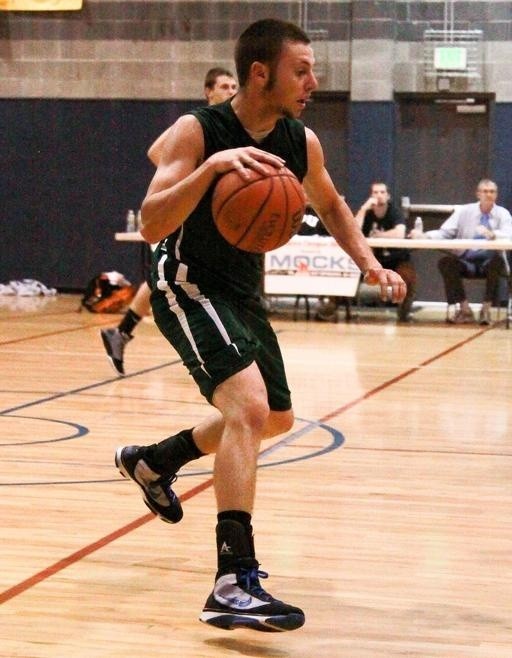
left=113, top=19, right=408, bottom=635
left=296, top=182, right=417, bottom=323
left=406, top=176, right=511, bottom=325
left=101, top=66, right=239, bottom=378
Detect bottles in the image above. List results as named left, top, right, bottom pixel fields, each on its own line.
left=414, top=217, right=424, bottom=239
left=126, top=209, right=142, bottom=233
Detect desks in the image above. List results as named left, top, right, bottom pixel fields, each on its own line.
left=114, top=230, right=512, bottom=319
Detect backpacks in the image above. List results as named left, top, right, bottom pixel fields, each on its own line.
left=83, top=269, right=133, bottom=313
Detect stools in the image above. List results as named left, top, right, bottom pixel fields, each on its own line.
left=443, top=273, right=506, bottom=324
left=288, top=290, right=353, bottom=322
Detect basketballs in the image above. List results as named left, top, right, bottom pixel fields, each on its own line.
left=211, top=163, right=306, bottom=253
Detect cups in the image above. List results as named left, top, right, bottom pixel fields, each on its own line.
left=401, top=195, right=409, bottom=204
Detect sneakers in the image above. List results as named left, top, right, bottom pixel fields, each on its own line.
left=99, top=325, right=135, bottom=376
left=199, top=559, right=303, bottom=633
left=446, top=307, right=491, bottom=325
left=115, top=444, right=183, bottom=524
left=396, top=310, right=410, bottom=323
left=314, top=304, right=336, bottom=321
